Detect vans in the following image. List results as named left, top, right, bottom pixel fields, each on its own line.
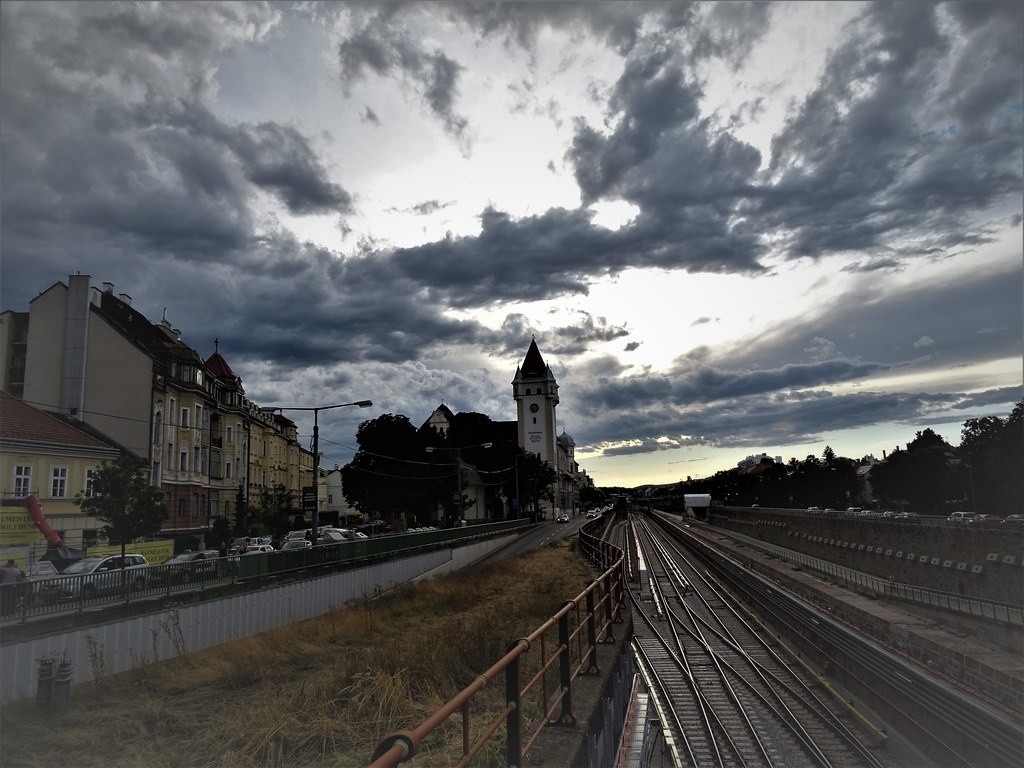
left=715, top=500, right=724, bottom=507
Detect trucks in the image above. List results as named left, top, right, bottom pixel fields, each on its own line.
left=454, top=520, right=466, bottom=528
left=684, top=494, right=717, bottom=508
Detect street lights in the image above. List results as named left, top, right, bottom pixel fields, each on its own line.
left=262, top=400, right=373, bottom=545
left=426, top=443, right=492, bottom=521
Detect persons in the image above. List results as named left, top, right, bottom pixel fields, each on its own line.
left=305, top=527, right=330, bottom=541
left=218, top=542, right=229, bottom=578
left=0, top=559, right=21, bottom=616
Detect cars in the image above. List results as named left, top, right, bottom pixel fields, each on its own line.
left=947, top=512, right=1024, bottom=531
left=150, top=520, right=397, bottom=587
left=860, top=510, right=920, bottom=520
left=805, top=507, right=834, bottom=513
left=586, top=507, right=600, bottom=518
left=557, top=513, right=570, bottom=522
left=752, top=504, right=760, bottom=511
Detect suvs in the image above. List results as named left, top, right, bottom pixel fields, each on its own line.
left=41, top=553, right=150, bottom=600
left=845, top=507, right=862, bottom=517
